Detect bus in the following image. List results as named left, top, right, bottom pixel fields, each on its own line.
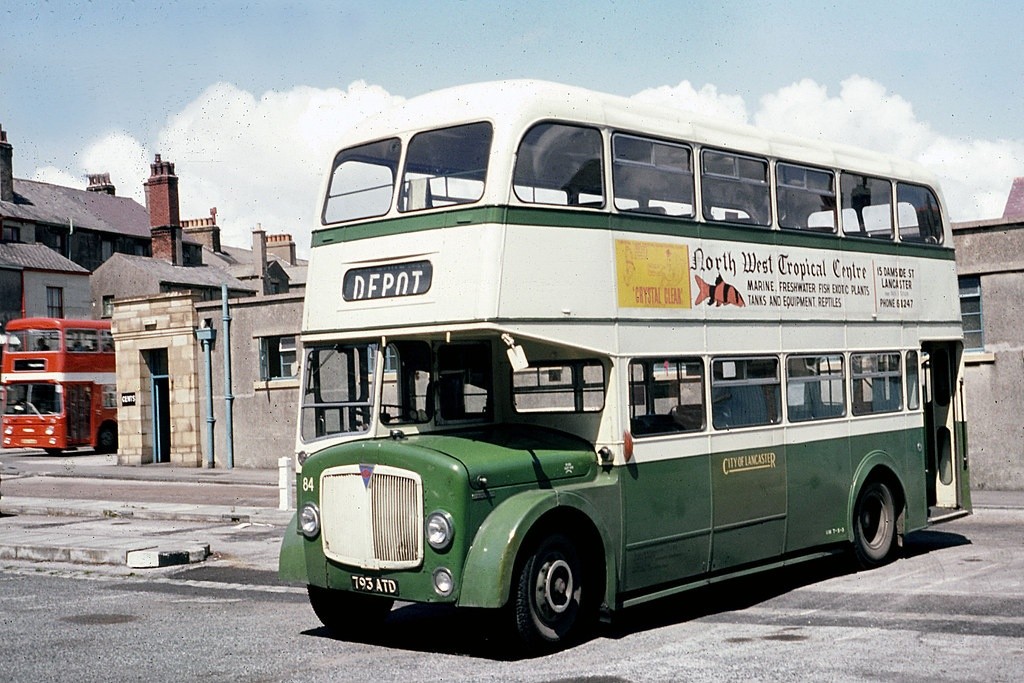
left=276, top=78, right=976, bottom=655
left=0, top=315, right=118, bottom=458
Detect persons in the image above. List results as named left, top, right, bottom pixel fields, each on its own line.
left=75, top=343, right=85, bottom=351
left=36, top=339, right=49, bottom=351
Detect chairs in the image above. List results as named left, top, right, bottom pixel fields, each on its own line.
left=637, top=416, right=686, bottom=434
left=426, top=363, right=466, bottom=421
left=821, top=401, right=847, bottom=417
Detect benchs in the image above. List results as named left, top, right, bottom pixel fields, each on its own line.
left=669, top=404, right=733, bottom=427
